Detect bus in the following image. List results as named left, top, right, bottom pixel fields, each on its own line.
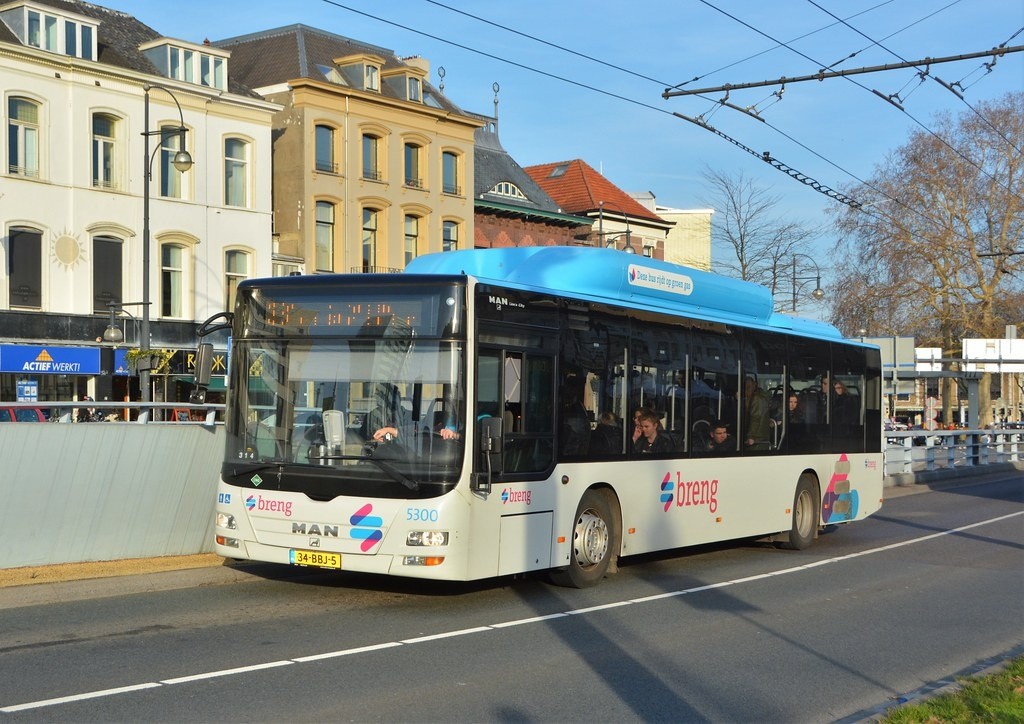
left=193, top=245, right=883, bottom=589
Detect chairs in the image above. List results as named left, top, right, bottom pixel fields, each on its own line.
left=657, top=384, right=863, bottom=450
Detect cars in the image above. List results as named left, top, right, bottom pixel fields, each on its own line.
left=912, top=429, right=942, bottom=446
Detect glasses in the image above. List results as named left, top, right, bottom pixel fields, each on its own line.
left=821, top=381, right=827, bottom=385
left=632, top=417, right=637, bottom=420
left=835, top=387, right=842, bottom=389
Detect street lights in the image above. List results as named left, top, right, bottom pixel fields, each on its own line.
left=791, top=253, right=825, bottom=312
left=859, top=327, right=868, bottom=343
left=104, top=83, right=197, bottom=403
left=597, top=200, right=635, bottom=254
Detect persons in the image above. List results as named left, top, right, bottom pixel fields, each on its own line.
left=358, top=383, right=467, bottom=444
left=597, top=369, right=855, bottom=454
